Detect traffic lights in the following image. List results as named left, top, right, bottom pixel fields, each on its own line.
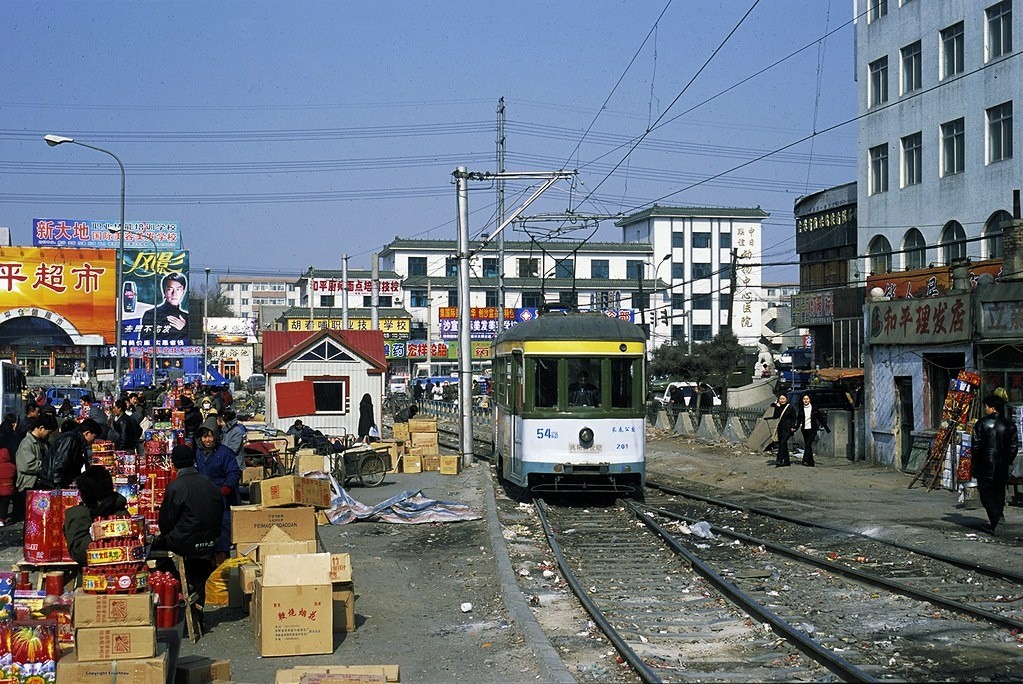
left=650, top=311, right=657, bottom=327
left=661, top=309, right=668, bottom=326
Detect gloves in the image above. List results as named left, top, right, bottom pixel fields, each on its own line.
left=220, top=486, right=230, bottom=496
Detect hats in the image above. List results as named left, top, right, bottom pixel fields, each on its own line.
left=180, top=394, right=194, bottom=408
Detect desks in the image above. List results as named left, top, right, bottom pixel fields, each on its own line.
left=16, top=549, right=204, bottom=645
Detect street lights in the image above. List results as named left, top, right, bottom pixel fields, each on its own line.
left=108, top=226, right=158, bottom=386
left=204, top=267, right=210, bottom=384
left=643, top=254, right=672, bottom=358
left=533, top=272, right=556, bottom=296
left=43, top=135, right=125, bottom=398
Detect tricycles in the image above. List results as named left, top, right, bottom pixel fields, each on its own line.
left=238, top=435, right=394, bottom=488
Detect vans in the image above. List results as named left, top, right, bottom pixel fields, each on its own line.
left=390, top=376, right=408, bottom=393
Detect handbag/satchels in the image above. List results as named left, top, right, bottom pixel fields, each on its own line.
left=771, top=425, right=779, bottom=442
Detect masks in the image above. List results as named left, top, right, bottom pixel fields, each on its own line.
left=203, top=403, right=211, bottom=408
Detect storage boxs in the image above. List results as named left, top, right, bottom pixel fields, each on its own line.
left=55, top=402, right=463, bottom=684
left=929, top=371, right=1023, bottom=492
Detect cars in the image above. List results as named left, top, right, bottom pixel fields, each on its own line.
left=770, top=387, right=849, bottom=410
left=247, top=376, right=265, bottom=392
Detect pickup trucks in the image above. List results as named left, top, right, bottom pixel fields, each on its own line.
left=654, top=381, right=721, bottom=406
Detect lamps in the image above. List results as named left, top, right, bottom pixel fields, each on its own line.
left=855, top=256, right=990, bottom=278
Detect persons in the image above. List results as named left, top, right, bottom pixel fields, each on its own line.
left=0, top=383, right=165, bottom=527
left=970, top=394, right=1018, bottom=537
left=180, top=385, right=234, bottom=439
left=568, top=371, right=599, bottom=406
left=774, top=394, right=796, bottom=467
left=669, top=385, right=686, bottom=413
left=140, top=272, right=189, bottom=340
left=793, top=393, right=831, bottom=467
left=194, top=416, right=240, bottom=563
left=153, top=444, right=224, bottom=632
left=357, top=394, right=375, bottom=442
left=394, top=405, right=418, bottom=423
left=472, top=378, right=492, bottom=396
left=414, top=380, right=454, bottom=402
left=287, top=420, right=305, bottom=439
left=64, top=465, right=131, bottom=573
left=218, top=411, right=246, bottom=506
left=690, top=383, right=713, bottom=414
left=761, top=364, right=771, bottom=378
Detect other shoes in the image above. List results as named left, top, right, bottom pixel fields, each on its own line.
left=783, top=462, right=790, bottom=466
left=994, top=517, right=1006, bottom=536
left=776, top=460, right=782, bottom=465
left=0, top=519, right=5, bottom=527
left=802, top=462, right=808, bottom=465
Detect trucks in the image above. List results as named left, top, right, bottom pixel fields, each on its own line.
left=45, top=387, right=101, bottom=416
left=120, top=373, right=152, bottom=390
left=774, top=346, right=812, bottom=389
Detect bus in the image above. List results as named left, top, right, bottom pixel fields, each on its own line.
left=489, top=315, right=646, bottom=493
left=412, top=360, right=493, bottom=387
left=2, top=361, right=29, bottom=426
